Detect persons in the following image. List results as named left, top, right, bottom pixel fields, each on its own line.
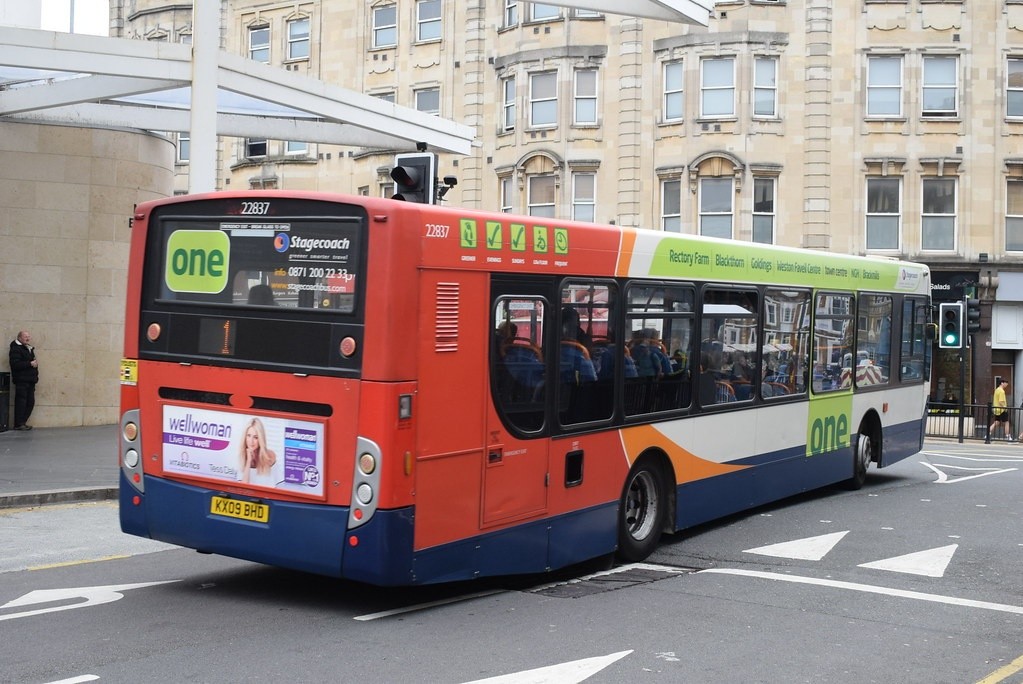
left=984, top=378, right=1013, bottom=441
left=813, top=362, right=819, bottom=391
left=236, top=415, right=281, bottom=487
left=688, top=350, right=793, bottom=403
left=495, top=307, right=689, bottom=377
left=247, top=285, right=274, bottom=307
left=9, top=330, right=39, bottom=430
left=1018, top=402, right=1023, bottom=441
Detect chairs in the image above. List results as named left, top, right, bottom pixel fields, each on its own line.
left=500, top=337, right=790, bottom=426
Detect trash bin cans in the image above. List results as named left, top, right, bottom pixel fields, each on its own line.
left=0, top=372, right=12, bottom=432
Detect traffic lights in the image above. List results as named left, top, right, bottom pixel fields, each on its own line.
left=387, top=151, right=440, bottom=207
left=938, top=303, right=962, bottom=349
left=965, top=294, right=982, bottom=348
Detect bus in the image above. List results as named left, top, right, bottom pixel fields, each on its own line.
left=115, top=187, right=938, bottom=586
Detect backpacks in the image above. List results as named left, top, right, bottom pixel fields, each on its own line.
left=651, top=351, right=664, bottom=372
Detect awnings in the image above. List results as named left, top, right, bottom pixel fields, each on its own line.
left=722, top=343, right=793, bottom=355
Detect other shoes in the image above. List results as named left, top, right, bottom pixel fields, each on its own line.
left=1005, top=434, right=1012, bottom=441
left=985, top=433, right=991, bottom=443
left=1017, top=437, right=1023, bottom=441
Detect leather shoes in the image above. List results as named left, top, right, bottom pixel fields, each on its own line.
left=14, top=425, right=32, bottom=431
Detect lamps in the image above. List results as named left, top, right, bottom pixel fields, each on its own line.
left=979, top=253, right=988, bottom=262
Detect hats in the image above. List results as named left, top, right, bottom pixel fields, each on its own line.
left=859, top=359, right=882, bottom=368
left=640, top=340, right=650, bottom=347
